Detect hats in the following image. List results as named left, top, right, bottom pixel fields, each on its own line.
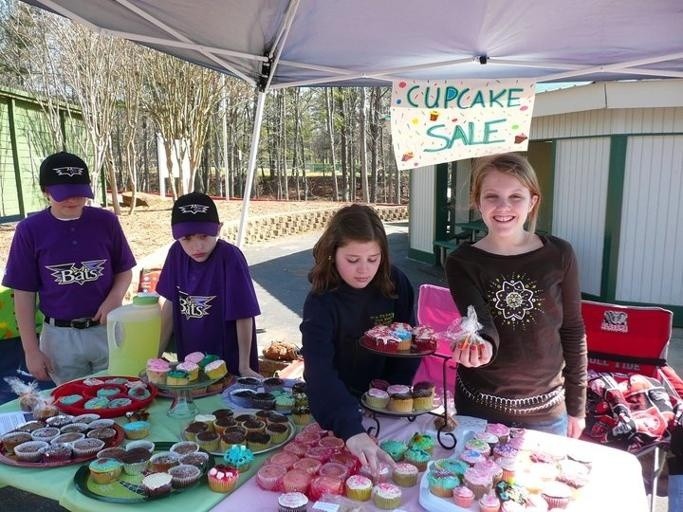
left=171, top=192, right=220, bottom=239
left=39, top=151, right=94, bottom=202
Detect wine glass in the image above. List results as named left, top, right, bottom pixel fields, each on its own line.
left=137, top=365, right=224, bottom=420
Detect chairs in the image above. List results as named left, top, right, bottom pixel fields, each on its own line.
left=409, top=281, right=462, bottom=404
left=578, top=296, right=682, bottom=511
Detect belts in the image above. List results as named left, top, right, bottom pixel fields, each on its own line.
left=44, top=315, right=99, bottom=329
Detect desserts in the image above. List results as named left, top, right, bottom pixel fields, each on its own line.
left=257, top=423, right=435, bottom=509
left=428, top=422, right=591, bottom=512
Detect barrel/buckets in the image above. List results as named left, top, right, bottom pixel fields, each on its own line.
left=105, top=293, right=163, bottom=377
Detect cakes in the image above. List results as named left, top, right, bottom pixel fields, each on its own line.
left=362, top=321, right=437, bottom=355
left=2, top=376, right=290, bottom=503
left=366, top=376, right=436, bottom=412
left=147, top=351, right=228, bottom=385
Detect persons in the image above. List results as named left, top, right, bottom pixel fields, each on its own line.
left=158, top=191, right=264, bottom=378
left=300, top=204, right=414, bottom=477
left=1, top=151, right=137, bottom=388
left=444, top=153, right=587, bottom=439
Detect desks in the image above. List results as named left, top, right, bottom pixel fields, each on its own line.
left=0, top=363, right=311, bottom=510
left=207, top=396, right=647, bottom=512
